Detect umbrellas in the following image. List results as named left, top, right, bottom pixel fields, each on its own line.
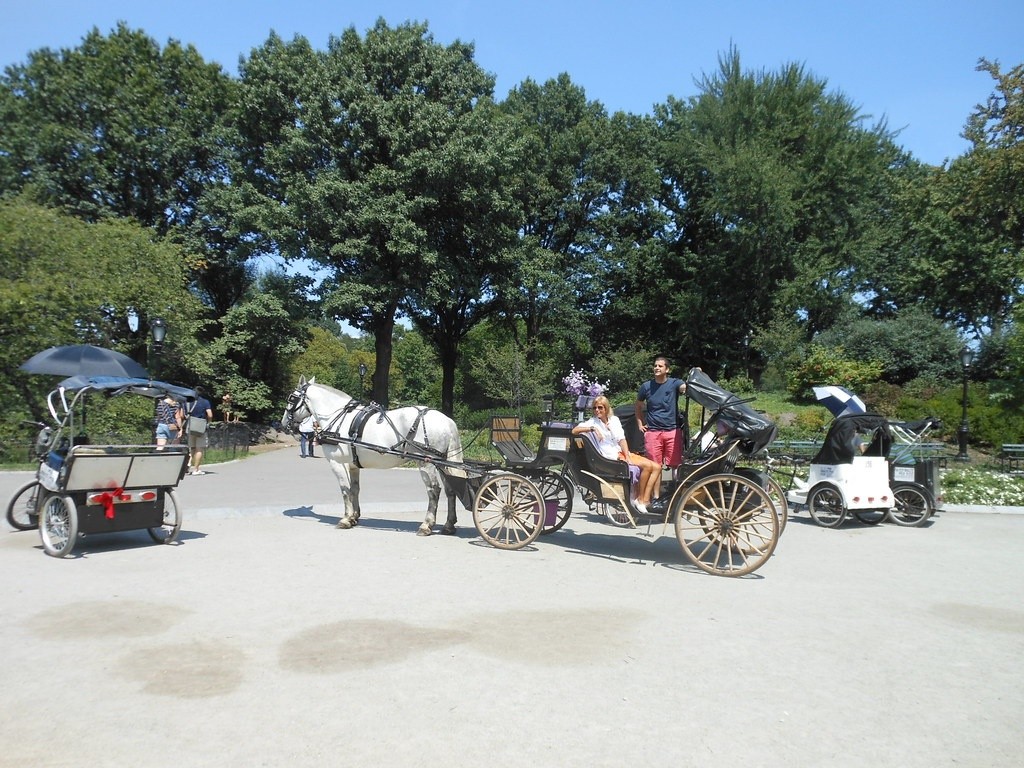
left=812, top=386, right=866, bottom=417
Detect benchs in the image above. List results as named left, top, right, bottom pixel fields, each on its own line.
left=51, top=443, right=189, bottom=493
left=579, top=434, right=728, bottom=491
left=999, top=444, right=1024, bottom=474
left=888, top=442, right=951, bottom=469
left=529, top=426, right=577, bottom=461
left=747, top=440, right=825, bottom=469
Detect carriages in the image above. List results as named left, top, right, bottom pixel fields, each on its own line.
left=280, top=368, right=789, bottom=578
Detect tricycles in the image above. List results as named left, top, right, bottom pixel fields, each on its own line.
left=760, top=411, right=954, bottom=531
left=5, top=339, right=197, bottom=559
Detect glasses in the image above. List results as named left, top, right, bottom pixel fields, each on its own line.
left=592, top=406, right=603, bottom=410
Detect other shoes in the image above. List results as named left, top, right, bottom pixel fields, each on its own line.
left=651, top=498, right=669, bottom=513
left=634, top=498, right=650, bottom=514
left=192, top=470, right=204, bottom=476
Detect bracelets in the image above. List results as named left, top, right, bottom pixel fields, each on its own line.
left=590, top=426, right=594, bottom=432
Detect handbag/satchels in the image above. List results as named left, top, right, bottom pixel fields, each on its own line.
left=168, top=424, right=178, bottom=430
left=189, top=416, right=207, bottom=436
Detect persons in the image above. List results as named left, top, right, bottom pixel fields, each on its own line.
left=635, top=358, right=686, bottom=508
left=299, top=415, right=319, bottom=458
left=157, top=387, right=212, bottom=475
left=689, top=421, right=725, bottom=453
left=852, top=431, right=916, bottom=464
left=572, top=396, right=661, bottom=512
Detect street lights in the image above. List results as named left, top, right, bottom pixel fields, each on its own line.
left=954, top=345, right=977, bottom=462
left=357, top=362, right=367, bottom=406
left=151, top=316, right=168, bottom=444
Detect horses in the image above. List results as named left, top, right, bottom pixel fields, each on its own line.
left=280, top=373, right=463, bottom=536
